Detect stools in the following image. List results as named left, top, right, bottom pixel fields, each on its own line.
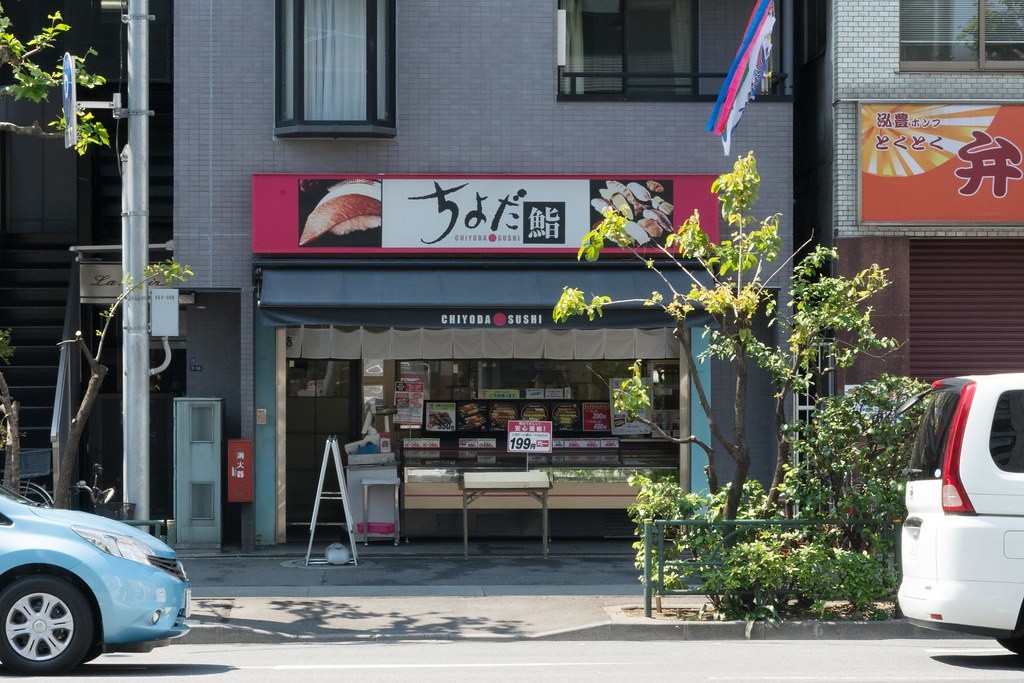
left=361, top=476, right=400, bottom=547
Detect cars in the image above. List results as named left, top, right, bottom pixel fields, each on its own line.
left=0, top=485, right=193, bottom=676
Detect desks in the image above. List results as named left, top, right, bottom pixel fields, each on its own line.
left=344, top=459, right=400, bottom=543
left=456, top=474, right=555, bottom=560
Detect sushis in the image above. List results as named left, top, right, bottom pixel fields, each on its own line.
left=590, top=179, right=674, bottom=249
left=298, top=178, right=382, bottom=249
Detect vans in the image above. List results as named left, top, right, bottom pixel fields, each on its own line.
left=898, top=372, right=1024, bottom=659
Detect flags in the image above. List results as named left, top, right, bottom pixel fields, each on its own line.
left=702, top=0, right=774, bottom=160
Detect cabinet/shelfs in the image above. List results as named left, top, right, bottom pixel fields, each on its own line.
left=398, top=432, right=680, bottom=545
